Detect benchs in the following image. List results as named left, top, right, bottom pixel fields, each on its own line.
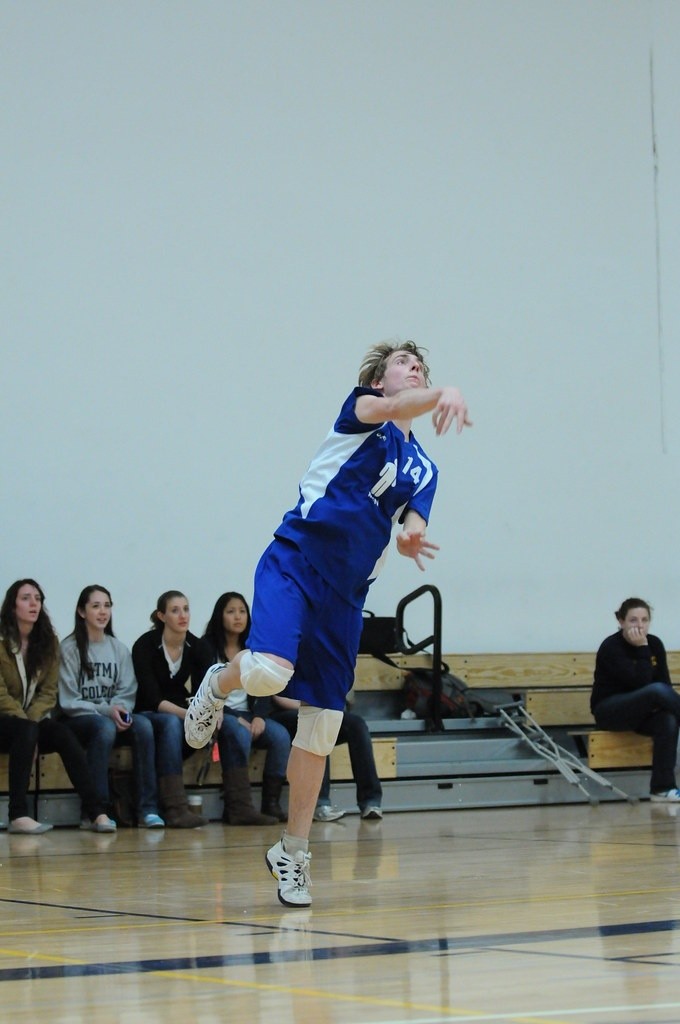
left=0, top=649, right=680, bottom=830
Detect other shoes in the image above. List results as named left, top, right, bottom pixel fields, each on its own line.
left=7, top=816, right=53, bottom=834
left=80, top=814, right=117, bottom=832
left=136, top=813, right=165, bottom=828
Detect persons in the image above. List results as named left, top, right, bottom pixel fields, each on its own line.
left=132, top=590, right=281, bottom=829
left=203, top=592, right=383, bottom=821
left=0, top=578, right=116, bottom=837
left=184, top=339, right=474, bottom=908
left=590, top=596, right=680, bottom=803
left=58, top=584, right=166, bottom=832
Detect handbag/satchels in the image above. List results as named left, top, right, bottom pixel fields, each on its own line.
left=398, top=668, right=502, bottom=718
left=357, top=610, right=405, bottom=653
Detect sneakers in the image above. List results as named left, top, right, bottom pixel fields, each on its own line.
left=649, top=789, right=680, bottom=803
left=312, top=805, right=346, bottom=821
left=265, top=841, right=313, bottom=907
left=185, top=661, right=234, bottom=748
left=360, top=806, right=384, bottom=820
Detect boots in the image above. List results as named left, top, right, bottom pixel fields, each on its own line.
left=261, top=774, right=290, bottom=822
left=159, top=773, right=210, bottom=828
left=220, top=767, right=265, bottom=825
